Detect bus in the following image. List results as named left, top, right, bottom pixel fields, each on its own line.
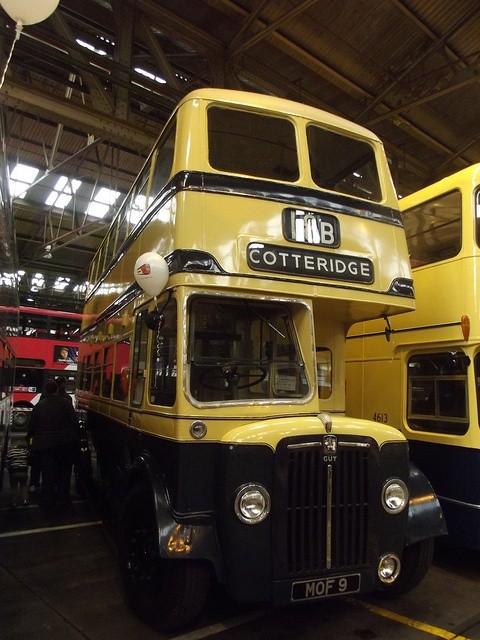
left=74, top=87, right=450, bottom=632
left=344, top=159, right=480, bottom=579
left=18, top=306, right=83, bottom=394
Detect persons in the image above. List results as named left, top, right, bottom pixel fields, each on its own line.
left=19, top=375, right=84, bottom=506
left=58, top=346, right=72, bottom=364
